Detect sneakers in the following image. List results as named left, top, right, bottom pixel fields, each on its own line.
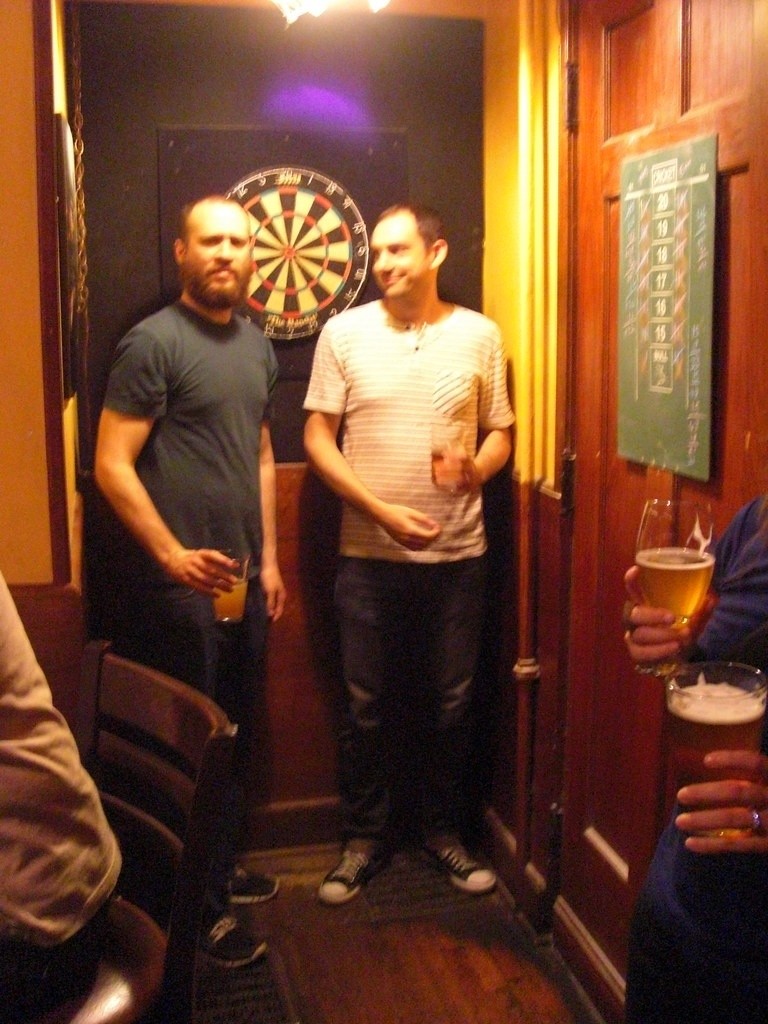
left=317, top=844, right=398, bottom=908
left=415, top=836, right=499, bottom=896
left=225, top=864, right=281, bottom=905
left=201, top=913, right=268, bottom=971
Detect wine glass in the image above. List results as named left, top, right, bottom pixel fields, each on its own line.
left=628, top=499, right=721, bottom=679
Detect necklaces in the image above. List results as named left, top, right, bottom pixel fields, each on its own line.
left=403, top=322, right=427, bottom=351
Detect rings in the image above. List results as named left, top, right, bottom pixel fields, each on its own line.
left=751, top=810, right=761, bottom=828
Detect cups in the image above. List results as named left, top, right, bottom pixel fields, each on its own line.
left=432, top=422, right=467, bottom=494
left=210, top=548, right=249, bottom=625
left=662, top=660, right=768, bottom=845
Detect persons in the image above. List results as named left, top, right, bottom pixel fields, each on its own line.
left=624, top=493, right=768, bottom=1024
left=302, top=206, right=515, bottom=903
left=92, top=196, right=287, bottom=969
left=0, top=573, right=124, bottom=1024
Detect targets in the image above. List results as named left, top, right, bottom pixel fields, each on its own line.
left=219, top=166, right=373, bottom=340
